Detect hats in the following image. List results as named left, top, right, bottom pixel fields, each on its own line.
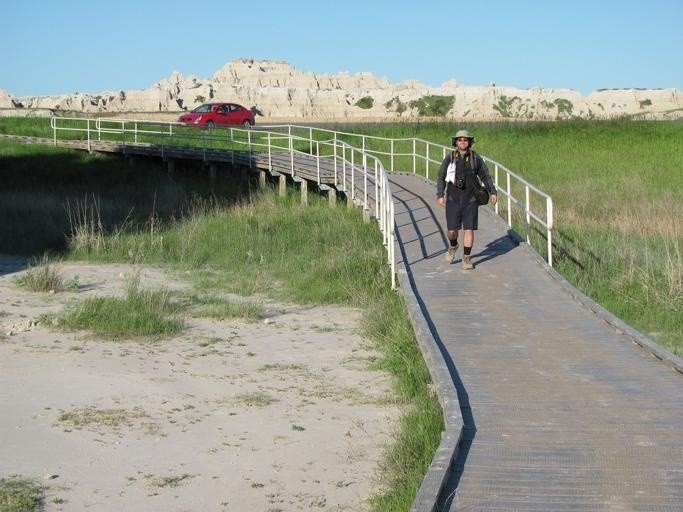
left=452, top=130, right=475, bottom=149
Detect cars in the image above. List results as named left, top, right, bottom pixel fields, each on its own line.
left=176, top=103, right=256, bottom=132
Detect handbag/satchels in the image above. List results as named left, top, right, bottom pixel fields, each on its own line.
left=474, top=186, right=489, bottom=205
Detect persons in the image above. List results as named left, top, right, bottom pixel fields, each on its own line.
left=437, top=130, right=497, bottom=269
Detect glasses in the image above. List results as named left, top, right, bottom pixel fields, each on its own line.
left=459, top=139, right=469, bottom=141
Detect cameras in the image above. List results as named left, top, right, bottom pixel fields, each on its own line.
left=456, top=178, right=465, bottom=190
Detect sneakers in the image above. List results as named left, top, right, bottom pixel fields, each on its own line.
left=446, top=242, right=459, bottom=264
left=461, top=255, right=473, bottom=270
